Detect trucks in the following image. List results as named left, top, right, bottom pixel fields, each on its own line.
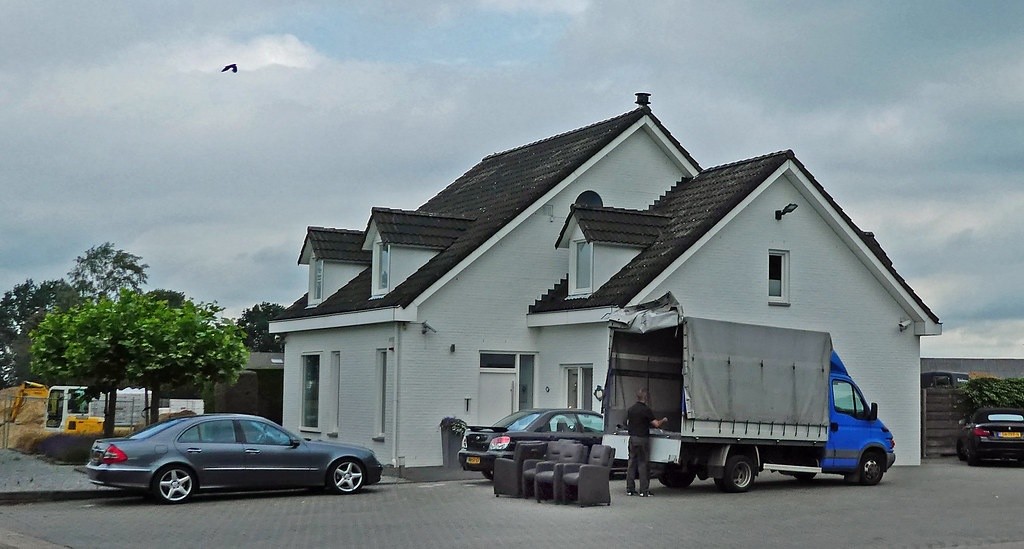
left=601, top=315, right=896, bottom=494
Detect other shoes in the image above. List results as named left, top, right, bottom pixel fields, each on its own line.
left=627, top=491, right=638, bottom=496
left=640, top=492, right=654, bottom=497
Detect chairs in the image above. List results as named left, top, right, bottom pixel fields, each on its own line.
left=213, top=426, right=236, bottom=443
left=494, top=439, right=616, bottom=508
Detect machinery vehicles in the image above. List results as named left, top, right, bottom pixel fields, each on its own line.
left=0, top=380, right=105, bottom=435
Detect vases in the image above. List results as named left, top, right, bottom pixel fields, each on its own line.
left=441, top=427, right=462, bottom=470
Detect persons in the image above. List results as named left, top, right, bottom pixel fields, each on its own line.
left=623, top=389, right=668, bottom=497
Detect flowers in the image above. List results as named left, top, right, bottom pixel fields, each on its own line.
left=439, top=416, right=466, bottom=435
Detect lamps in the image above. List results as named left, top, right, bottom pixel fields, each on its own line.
left=594, top=384, right=604, bottom=402
left=450, top=344, right=455, bottom=352
left=775, top=203, right=798, bottom=220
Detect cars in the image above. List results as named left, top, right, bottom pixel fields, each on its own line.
left=457, top=407, right=603, bottom=481
left=85, top=413, right=385, bottom=506
left=955, top=406, right=1024, bottom=467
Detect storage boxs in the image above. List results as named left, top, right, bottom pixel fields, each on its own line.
left=997, top=432, right=1021, bottom=438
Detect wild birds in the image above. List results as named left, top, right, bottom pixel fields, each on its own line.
left=221, top=64, right=238, bottom=74
